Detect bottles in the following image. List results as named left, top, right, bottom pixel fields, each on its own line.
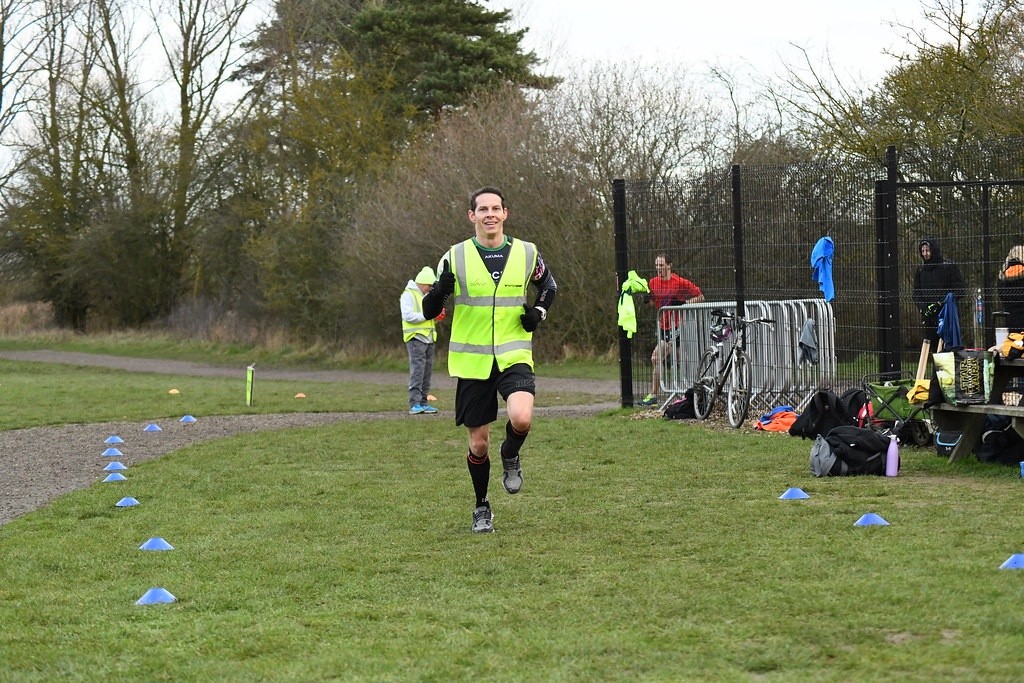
left=886, top=435, right=899, bottom=477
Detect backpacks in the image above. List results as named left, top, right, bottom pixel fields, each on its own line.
left=788, top=388, right=867, bottom=442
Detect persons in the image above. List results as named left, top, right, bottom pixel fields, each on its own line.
left=400, top=266, right=445, bottom=414
left=422, top=187, right=557, bottom=535
left=997, top=246, right=1024, bottom=334
left=912, top=240, right=963, bottom=354
left=638, top=253, right=706, bottom=406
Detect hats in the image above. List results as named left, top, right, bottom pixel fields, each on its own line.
left=414, top=265, right=436, bottom=286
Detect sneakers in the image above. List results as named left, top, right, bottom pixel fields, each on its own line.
left=408, top=404, right=425, bottom=414
left=635, top=392, right=659, bottom=407
left=422, top=406, right=438, bottom=414
left=471, top=505, right=495, bottom=536
left=501, top=440, right=524, bottom=495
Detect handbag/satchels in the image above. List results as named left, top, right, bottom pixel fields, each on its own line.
left=931, top=345, right=1000, bottom=407
left=808, top=426, right=901, bottom=477
left=933, top=430, right=964, bottom=456
left=663, top=386, right=696, bottom=419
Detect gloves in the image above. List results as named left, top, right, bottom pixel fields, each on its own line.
left=919, top=304, right=941, bottom=324
left=520, top=302, right=544, bottom=332
left=436, top=259, right=455, bottom=295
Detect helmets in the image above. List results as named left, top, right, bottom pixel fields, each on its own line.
left=709, top=321, right=732, bottom=342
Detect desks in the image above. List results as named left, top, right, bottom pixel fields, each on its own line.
left=945, top=355, right=1024, bottom=470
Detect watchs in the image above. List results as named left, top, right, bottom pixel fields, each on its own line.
left=534, top=306, right=546, bottom=321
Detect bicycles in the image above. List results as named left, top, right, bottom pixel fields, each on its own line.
left=693, top=308, right=775, bottom=430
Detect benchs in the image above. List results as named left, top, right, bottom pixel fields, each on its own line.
left=912, top=401, right=1024, bottom=418
